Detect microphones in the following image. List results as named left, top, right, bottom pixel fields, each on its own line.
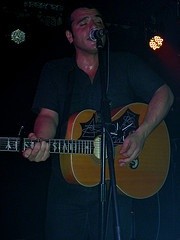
left=89, top=27, right=111, bottom=42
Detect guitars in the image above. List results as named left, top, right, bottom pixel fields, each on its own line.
left=0, top=102, right=171, bottom=200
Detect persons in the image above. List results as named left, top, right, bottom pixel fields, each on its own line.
left=23, top=2, right=175, bottom=240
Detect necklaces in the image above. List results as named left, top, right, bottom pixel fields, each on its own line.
left=78, top=63, right=98, bottom=70
